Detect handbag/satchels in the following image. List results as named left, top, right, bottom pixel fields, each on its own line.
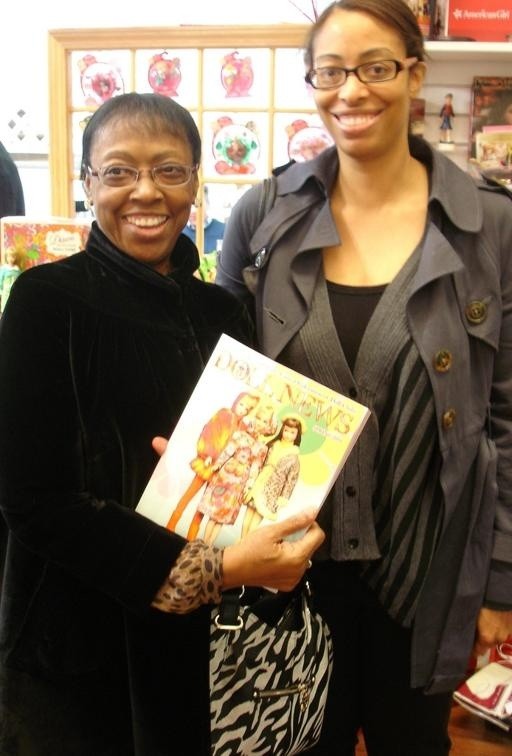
left=198, top=583, right=334, bottom=756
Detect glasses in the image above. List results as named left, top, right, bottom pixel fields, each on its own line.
left=81, top=161, right=200, bottom=188
left=304, top=55, right=419, bottom=90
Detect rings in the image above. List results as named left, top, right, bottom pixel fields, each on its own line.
left=307, top=559, right=312, bottom=569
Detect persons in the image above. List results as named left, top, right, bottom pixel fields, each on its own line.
left=167, top=392, right=305, bottom=553
left=207, top=1, right=510, bottom=753
left=0, top=90, right=329, bottom=756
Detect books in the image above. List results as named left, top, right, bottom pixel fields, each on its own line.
left=136, top=333, right=371, bottom=592
